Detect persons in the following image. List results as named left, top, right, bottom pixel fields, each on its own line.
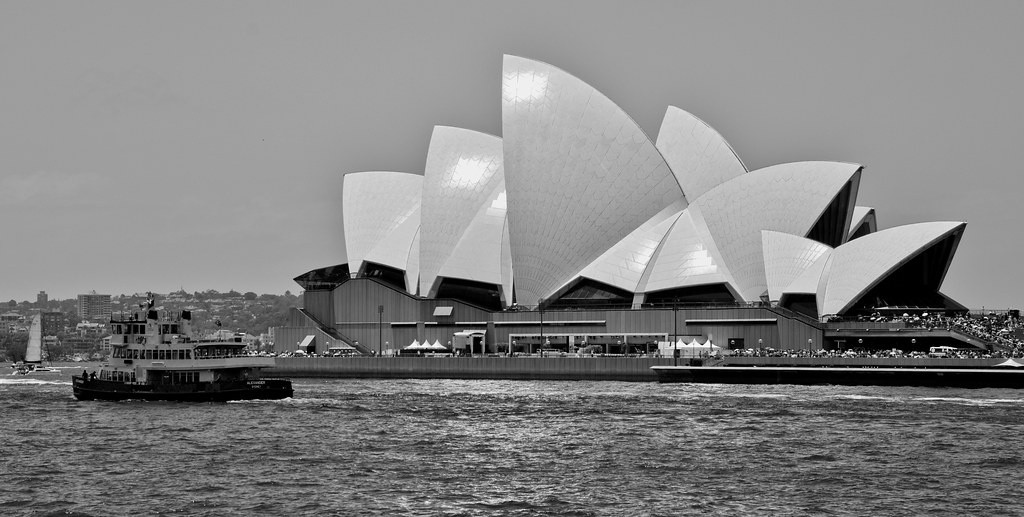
left=82, top=370, right=96, bottom=382
left=733, top=300, right=1024, bottom=359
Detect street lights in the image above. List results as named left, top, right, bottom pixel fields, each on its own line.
left=326, top=342, right=329, bottom=351
left=808, top=339, right=812, bottom=357
left=672, top=296, right=680, bottom=366
left=448, top=341, right=452, bottom=358
left=912, top=339, right=916, bottom=358
left=480, top=341, right=483, bottom=357
left=297, top=342, right=300, bottom=350
left=759, top=339, right=762, bottom=357
left=859, top=338, right=862, bottom=357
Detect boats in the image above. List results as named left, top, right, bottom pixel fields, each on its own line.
left=73, top=354, right=82, bottom=362
left=71, top=299, right=295, bottom=403
left=92, top=353, right=105, bottom=361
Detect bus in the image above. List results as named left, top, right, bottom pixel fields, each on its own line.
left=324, top=348, right=356, bottom=357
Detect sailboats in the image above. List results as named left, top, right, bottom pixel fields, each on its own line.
left=11, top=312, right=61, bottom=376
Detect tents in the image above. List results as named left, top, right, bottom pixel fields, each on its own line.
left=403, top=339, right=448, bottom=358
left=664, top=338, right=721, bottom=358
